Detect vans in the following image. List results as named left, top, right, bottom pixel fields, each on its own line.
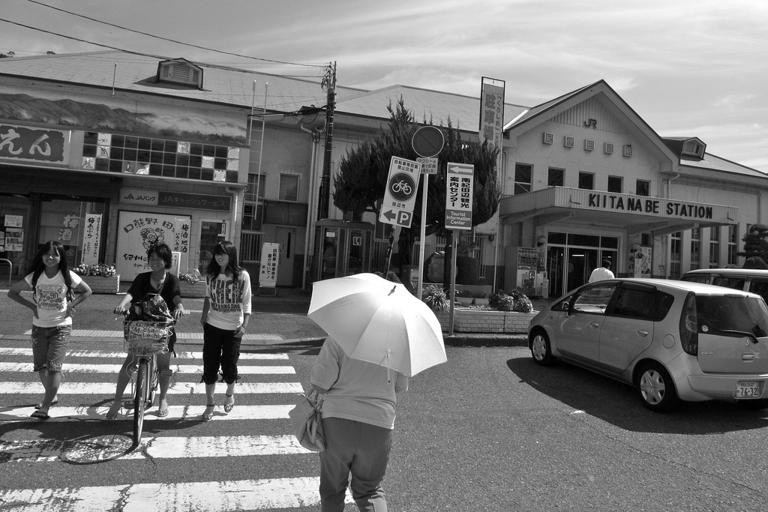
left=680, top=266, right=768, bottom=302
left=527, top=276, right=768, bottom=412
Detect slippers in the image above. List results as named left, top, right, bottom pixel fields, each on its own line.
left=158, top=402, right=168, bottom=416
left=107, top=404, right=121, bottom=419
left=31, top=411, right=50, bottom=419
left=36, top=399, right=58, bottom=408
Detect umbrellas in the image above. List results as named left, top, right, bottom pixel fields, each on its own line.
left=307, top=272, right=448, bottom=383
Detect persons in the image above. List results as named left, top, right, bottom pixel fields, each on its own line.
left=309, top=334, right=409, bottom=511
left=7, top=240, right=93, bottom=420
left=587, top=259, right=616, bottom=304
left=199, top=240, right=253, bottom=421
left=106, top=243, right=186, bottom=421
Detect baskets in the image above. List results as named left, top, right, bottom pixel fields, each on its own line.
left=122, top=321, right=170, bottom=357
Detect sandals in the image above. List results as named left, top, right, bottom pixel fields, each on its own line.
left=202, top=404, right=215, bottom=420
left=224, top=394, right=235, bottom=412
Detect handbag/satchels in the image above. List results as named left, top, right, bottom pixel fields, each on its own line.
left=289, top=388, right=326, bottom=453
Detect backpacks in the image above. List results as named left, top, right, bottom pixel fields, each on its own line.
left=123, top=292, right=176, bottom=359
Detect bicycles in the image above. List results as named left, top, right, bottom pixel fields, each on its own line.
left=109, top=305, right=185, bottom=447
